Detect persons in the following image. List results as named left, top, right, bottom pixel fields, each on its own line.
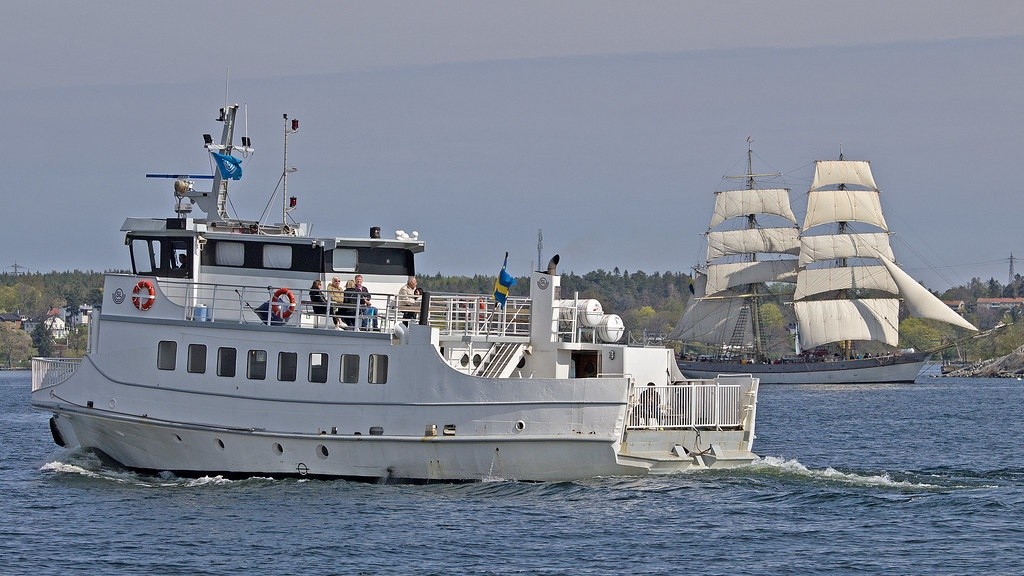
left=309, top=280, right=343, bottom=330
left=696, top=357, right=708, bottom=362
left=179, top=254, right=186, bottom=268
left=327, top=275, right=380, bottom=331
left=635, top=382, right=663, bottom=430
left=850, top=350, right=871, bottom=359
left=887, top=350, right=890, bottom=355
left=690, top=357, right=693, bottom=361
left=399, top=276, right=423, bottom=327
left=763, top=357, right=771, bottom=364
left=876, top=353, right=879, bottom=357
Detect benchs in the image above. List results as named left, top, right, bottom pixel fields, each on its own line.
left=301, top=301, right=379, bottom=331
left=380, top=305, right=450, bottom=331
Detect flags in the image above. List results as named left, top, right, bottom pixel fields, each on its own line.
left=492, top=258, right=517, bottom=310
left=210, top=151, right=243, bottom=181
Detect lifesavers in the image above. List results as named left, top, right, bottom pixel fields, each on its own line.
left=270, top=287, right=296, bottom=319
left=460, top=296, right=485, bottom=323
left=131, top=279, right=157, bottom=312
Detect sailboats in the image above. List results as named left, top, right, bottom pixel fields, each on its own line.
left=662, top=136, right=1014, bottom=384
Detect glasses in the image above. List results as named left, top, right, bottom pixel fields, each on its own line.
left=334, top=280, right=340, bottom=282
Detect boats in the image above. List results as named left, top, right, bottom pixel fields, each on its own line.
left=30, top=66, right=762, bottom=485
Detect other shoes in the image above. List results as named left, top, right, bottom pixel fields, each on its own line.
left=340, top=322, right=347, bottom=327
left=334, top=327, right=344, bottom=331
